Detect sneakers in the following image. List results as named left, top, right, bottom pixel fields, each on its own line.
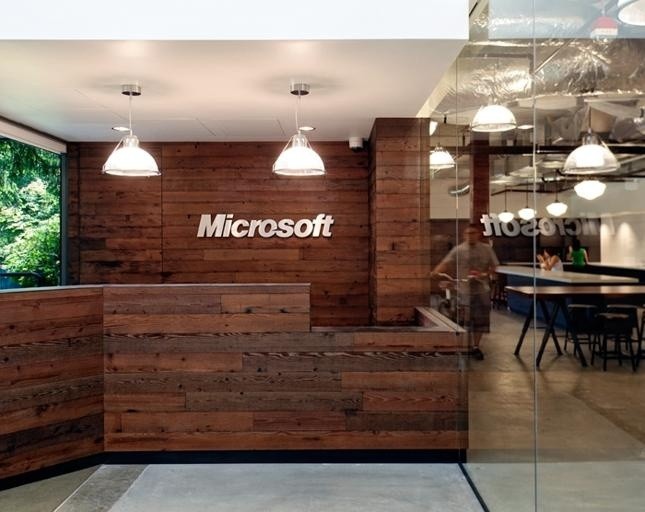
left=471, top=348, right=483, bottom=360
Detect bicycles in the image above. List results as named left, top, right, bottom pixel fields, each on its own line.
left=436, top=271, right=491, bottom=355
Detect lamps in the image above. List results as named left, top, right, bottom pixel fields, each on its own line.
left=99, top=84, right=327, bottom=177
left=427, top=101, right=619, bottom=222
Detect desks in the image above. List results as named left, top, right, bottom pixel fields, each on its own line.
left=492, top=261, right=645, bottom=373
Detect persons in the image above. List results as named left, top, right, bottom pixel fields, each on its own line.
left=537, top=246, right=564, bottom=274
left=432, top=222, right=500, bottom=361
left=567, top=238, right=589, bottom=266
